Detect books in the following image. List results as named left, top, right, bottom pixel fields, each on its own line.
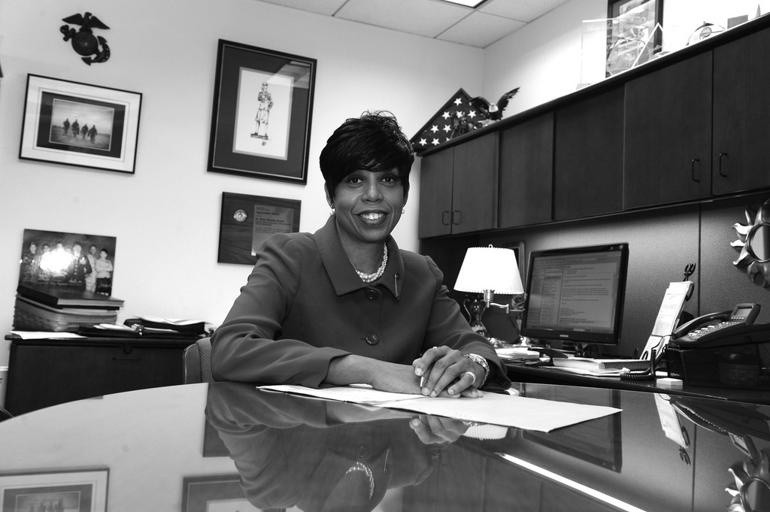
left=16, top=286, right=128, bottom=311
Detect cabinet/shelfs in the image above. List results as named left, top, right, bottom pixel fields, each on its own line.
left=623, top=12, right=770, bottom=217
left=499, top=75, right=622, bottom=230
left=420, top=123, right=500, bottom=239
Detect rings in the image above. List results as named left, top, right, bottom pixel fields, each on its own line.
left=468, top=370, right=477, bottom=386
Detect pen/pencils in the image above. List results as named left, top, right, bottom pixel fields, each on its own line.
left=420, top=346, right=437, bottom=390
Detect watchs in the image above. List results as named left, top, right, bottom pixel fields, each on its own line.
left=464, top=351, right=490, bottom=389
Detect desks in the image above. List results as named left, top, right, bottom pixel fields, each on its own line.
left=0, top=382, right=770, bottom=512
left=4, top=334, right=198, bottom=415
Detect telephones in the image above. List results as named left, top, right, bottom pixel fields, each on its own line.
left=669, top=303, right=770, bottom=348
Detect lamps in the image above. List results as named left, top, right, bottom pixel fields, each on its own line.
left=454, top=244, right=525, bottom=336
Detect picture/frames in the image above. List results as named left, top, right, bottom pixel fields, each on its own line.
left=217, top=191, right=301, bottom=266
left=0, top=468, right=111, bottom=512
left=181, top=475, right=263, bottom=512
left=605, top=0, right=665, bottom=77
left=18, top=73, right=143, bottom=174
left=207, top=38, right=318, bottom=186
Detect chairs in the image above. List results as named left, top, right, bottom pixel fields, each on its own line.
left=184, top=337, right=212, bottom=384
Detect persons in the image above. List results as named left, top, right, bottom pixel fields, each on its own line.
left=209, top=109, right=511, bottom=401
left=62, top=119, right=96, bottom=143
left=206, top=379, right=472, bottom=512
left=24, top=243, right=112, bottom=295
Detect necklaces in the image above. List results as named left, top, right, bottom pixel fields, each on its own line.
left=344, top=460, right=374, bottom=503
left=348, top=241, right=387, bottom=283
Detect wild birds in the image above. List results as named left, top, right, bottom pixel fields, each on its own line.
left=467, top=87, right=520, bottom=122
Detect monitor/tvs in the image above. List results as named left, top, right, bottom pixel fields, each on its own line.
left=520, top=242, right=629, bottom=354
left=519, top=383, right=624, bottom=474
